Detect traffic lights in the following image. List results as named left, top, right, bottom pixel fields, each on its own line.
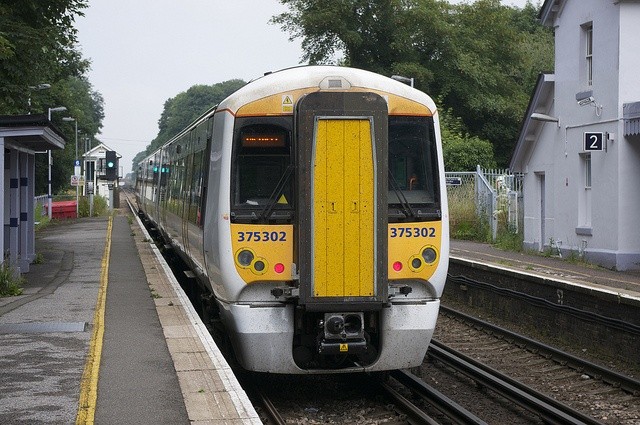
left=106, top=151, right=117, bottom=180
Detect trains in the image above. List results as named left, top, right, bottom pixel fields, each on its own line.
left=136, top=65, right=450, bottom=399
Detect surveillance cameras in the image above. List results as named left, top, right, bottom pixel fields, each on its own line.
left=578, top=96, right=595, bottom=108
